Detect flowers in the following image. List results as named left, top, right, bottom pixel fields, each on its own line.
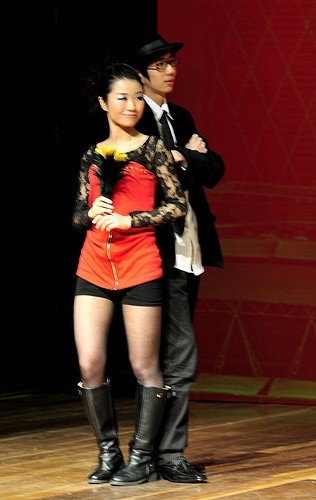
left=86, top=145, right=132, bottom=215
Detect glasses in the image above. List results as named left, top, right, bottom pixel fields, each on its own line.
left=147, top=59, right=180, bottom=71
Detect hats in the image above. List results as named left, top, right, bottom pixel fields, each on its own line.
left=133, top=33, right=185, bottom=72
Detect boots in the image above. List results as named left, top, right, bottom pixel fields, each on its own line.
left=109, top=384, right=173, bottom=486
left=78, top=380, right=128, bottom=487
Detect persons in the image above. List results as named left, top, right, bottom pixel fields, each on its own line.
left=72, top=62, right=187, bottom=487
left=129, top=31, right=225, bottom=482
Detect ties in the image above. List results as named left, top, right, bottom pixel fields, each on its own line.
left=157, top=111, right=186, bottom=237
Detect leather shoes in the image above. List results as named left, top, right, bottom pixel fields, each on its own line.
left=161, top=457, right=210, bottom=485
